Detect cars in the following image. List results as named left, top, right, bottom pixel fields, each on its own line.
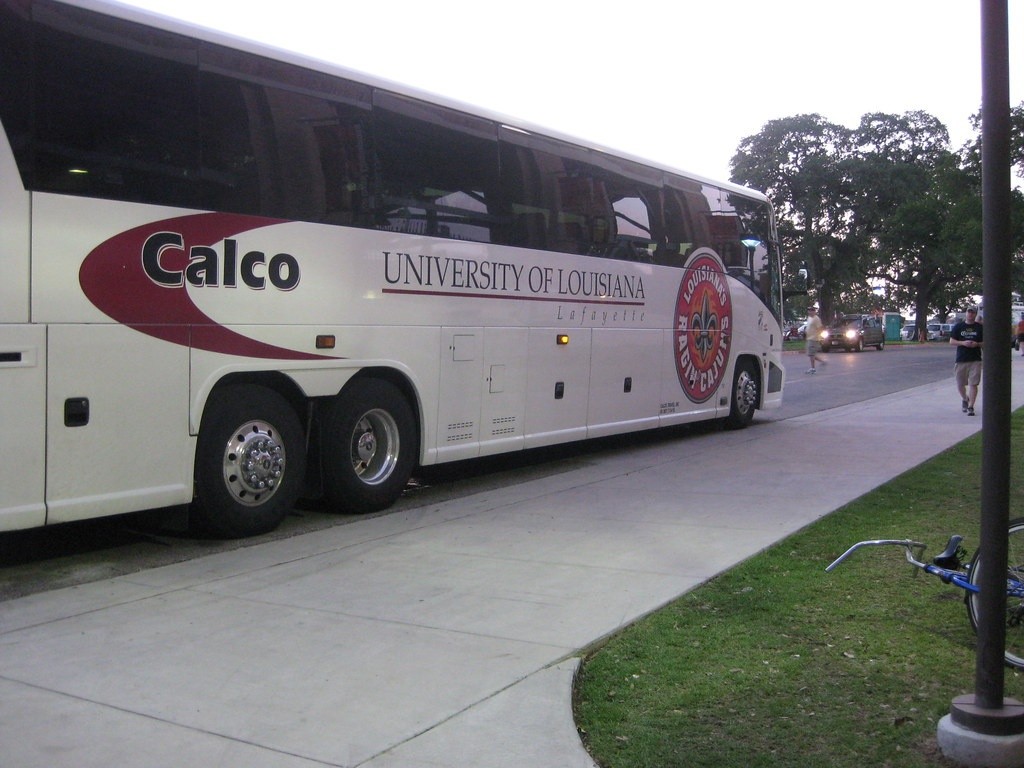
left=898, top=324, right=956, bottom=341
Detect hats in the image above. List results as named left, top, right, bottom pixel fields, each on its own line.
left=967, top=306, right=978, bottom=313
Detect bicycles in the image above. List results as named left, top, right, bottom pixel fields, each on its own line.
left=823, top=516, right=1024, bottom=674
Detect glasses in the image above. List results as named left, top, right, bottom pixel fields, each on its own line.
left=807, top=308, right=814, bottom=311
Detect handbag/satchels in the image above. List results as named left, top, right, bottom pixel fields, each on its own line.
left=1015, top=340, right=1019, bottom=351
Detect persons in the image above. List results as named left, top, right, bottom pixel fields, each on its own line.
left=949, top=306, right=984, bottom=416
left=804, top=307, right=826, bottom=376
left=1016, top=314, right=1024, bottom=357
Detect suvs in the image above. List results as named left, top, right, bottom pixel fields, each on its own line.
left=785, top=321, right=810, bottom=340
left=819, top=314, right=884, bottom=351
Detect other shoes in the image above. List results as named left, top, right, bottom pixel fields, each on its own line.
left=967, top=407, right=975, bottom=416
left=805, top=369, right=817, bottom=374
left=822, top=359, right=826, bottom=365
left=962, top=396, right=969, bottom=412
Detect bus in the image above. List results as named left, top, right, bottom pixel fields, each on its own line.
left=0, top=0, right=813, bottom=544
